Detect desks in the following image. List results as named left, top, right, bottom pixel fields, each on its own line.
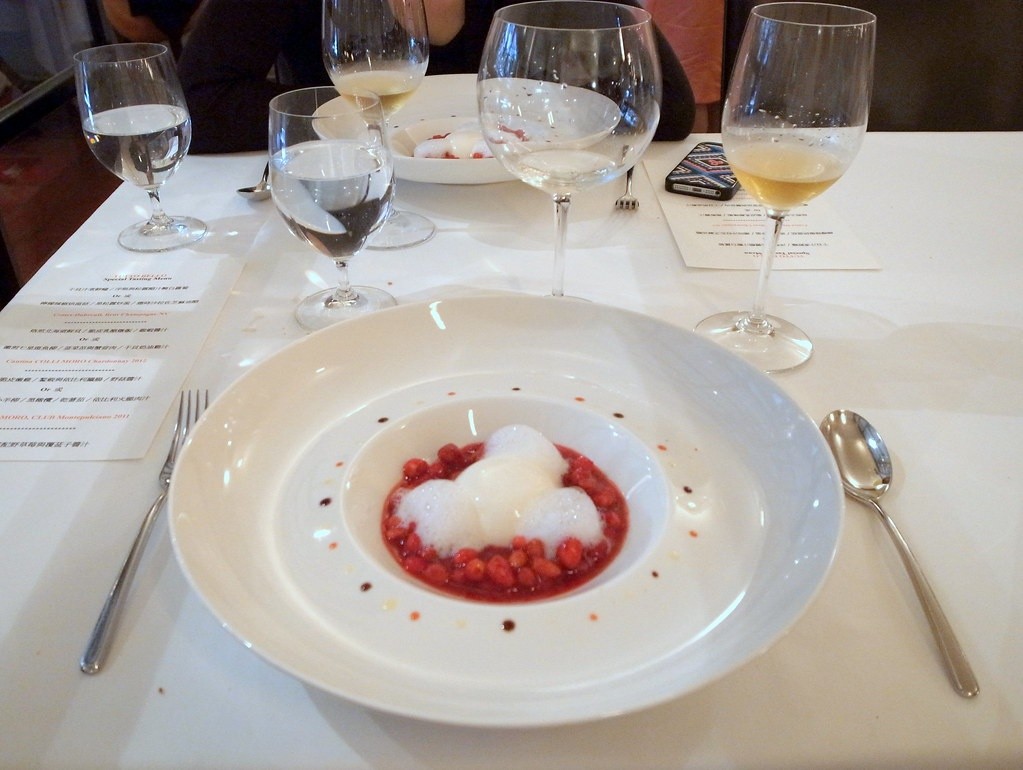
left=0, top=132, right=1023, bottom=770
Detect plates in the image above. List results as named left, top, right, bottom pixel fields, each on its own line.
left=311, top=73, right=621, bottom=185
left=168, top=293, right=847, bottom=729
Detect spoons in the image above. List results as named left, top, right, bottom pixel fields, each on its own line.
left=818, top=410, right=980, bottom=699
left=237, top=162, right=272, bottom=201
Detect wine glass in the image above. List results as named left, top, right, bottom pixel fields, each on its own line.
left=74, top=42, right=206, bottom=251
left=694, top=2, right=875, bottom=371
left=322, top=0, right=436, bottom=249
left=476, top=0, right=662, bottom=301
left=268, top=85, right=397, bottom=331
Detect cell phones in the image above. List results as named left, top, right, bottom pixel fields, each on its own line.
left=666, top=143, right=742, bottom=200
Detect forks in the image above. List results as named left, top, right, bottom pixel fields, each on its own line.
left=81, top=388, right=208, bottom=675
left=615, top=146, right=639, bottom=211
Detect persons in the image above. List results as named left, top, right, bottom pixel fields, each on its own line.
left=174, top=0, right=695, bottom=155
